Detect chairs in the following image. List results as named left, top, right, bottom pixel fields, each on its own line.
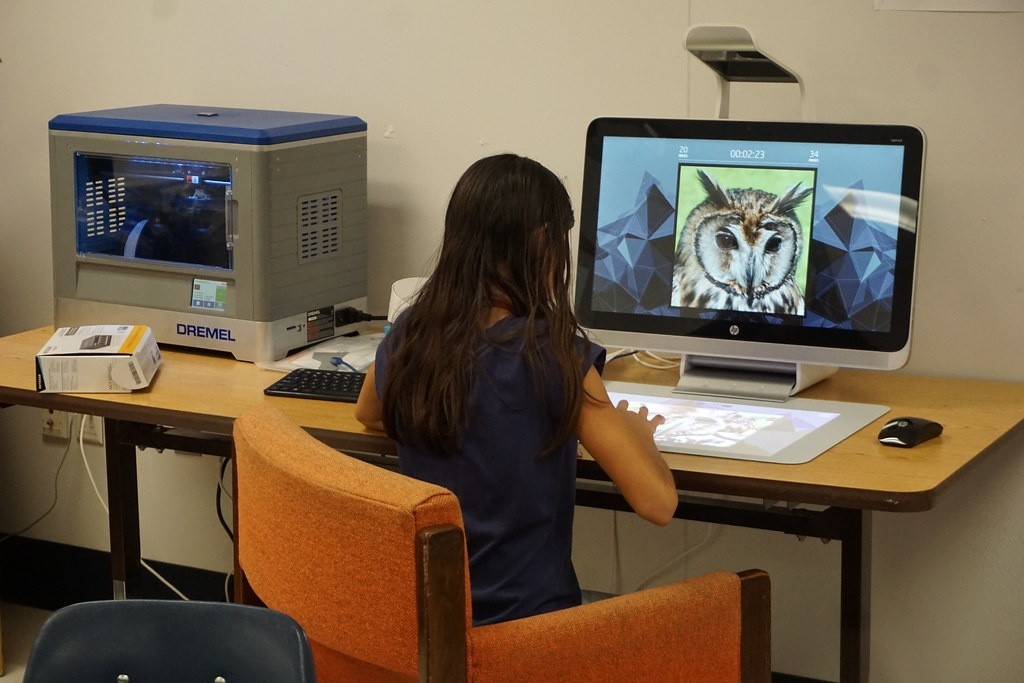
left=22, top=598, right=319, bottom=683
left=232, top=408, right=773, bottom=682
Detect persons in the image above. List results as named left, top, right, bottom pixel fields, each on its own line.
left=354, top=154, right=679, bottom=627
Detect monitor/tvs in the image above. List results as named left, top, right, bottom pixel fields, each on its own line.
left=572, top=116, right=926, bottom=404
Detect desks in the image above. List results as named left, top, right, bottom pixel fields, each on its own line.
left=0, top=313, right=1024, bottom=683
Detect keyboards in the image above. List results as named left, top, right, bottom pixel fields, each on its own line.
left=264, top=368, right=369, bottom=403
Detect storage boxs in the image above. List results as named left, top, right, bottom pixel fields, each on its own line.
left=33, top=325, right=165, bottom=393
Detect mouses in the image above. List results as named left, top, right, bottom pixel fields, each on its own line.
left=876, top=416, right=944, bottom=449
